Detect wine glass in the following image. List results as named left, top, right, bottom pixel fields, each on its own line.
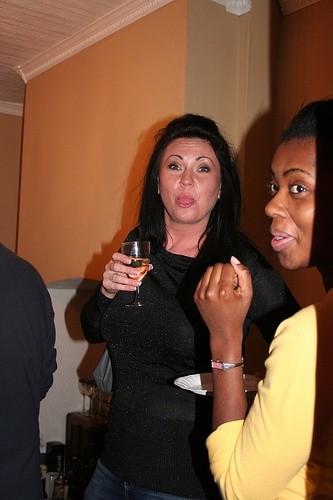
left=78, top=377, right=107, bottom=423
left=119, top=241, right=153, bottom=308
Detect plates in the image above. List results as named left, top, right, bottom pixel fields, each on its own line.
left=175, top=373, right=262, bottom=395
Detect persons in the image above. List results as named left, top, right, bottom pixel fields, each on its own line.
left=81, top=111, right=306, bottom=500
left=0, top=237, right=58, bottom=500
left=193, top=95, right=333, bottom=500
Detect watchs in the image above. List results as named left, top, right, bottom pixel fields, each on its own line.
left=210, top=356, right=244, bottom=370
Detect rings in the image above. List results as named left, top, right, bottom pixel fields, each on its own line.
left=111, top=273, right=118, bottom=283
